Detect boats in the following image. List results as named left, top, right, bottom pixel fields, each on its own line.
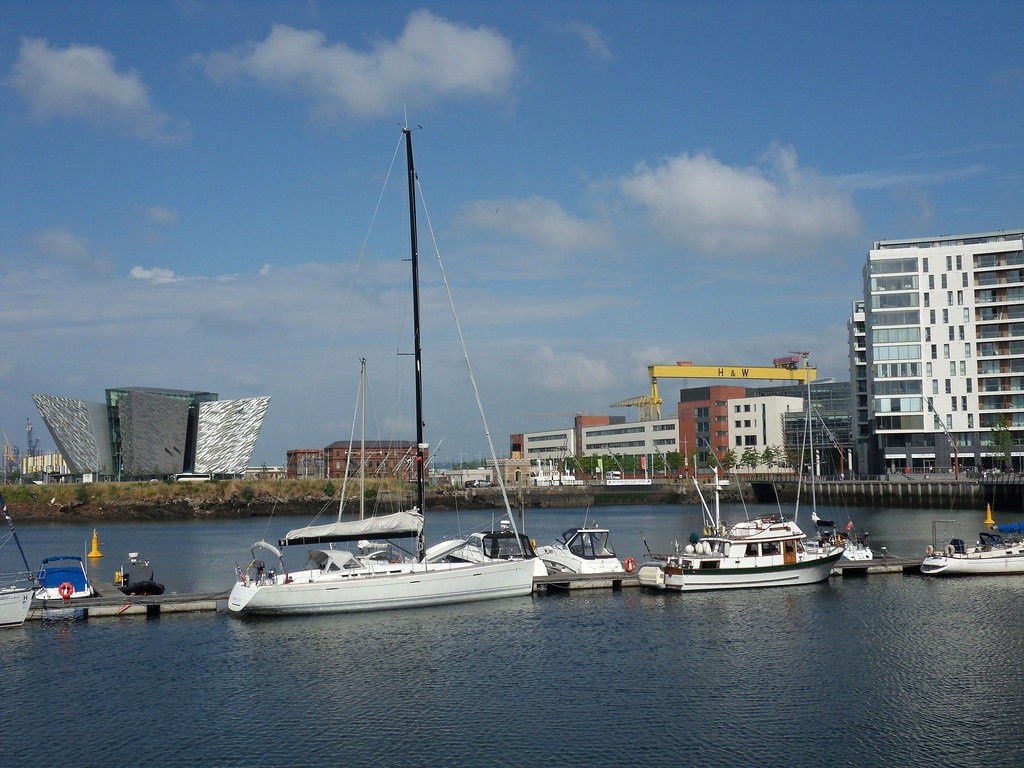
left=445, top=491, right=549, bottom=592
left=0, top=568, right=47, bottom=628
left=114, top=552, right=165, bottom=595
left=920, top=519, right=1024, bottom=574
left=35, top=555, right=94, bottom=600
left=533, top=502, right=626, bottom=582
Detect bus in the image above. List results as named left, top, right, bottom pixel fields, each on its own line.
left=171, top=473, right=211, bottom=483
left=606, top=471, right=621, bottom=480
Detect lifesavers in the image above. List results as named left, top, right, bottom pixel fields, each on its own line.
left=926, top=545, right=933, bottom=555
left=944, top=544, right=955, bottom=555
left=58, top=583, right=73, bottom=598
left=530, top=540, right=536, bottom=550
left=625, top=557, right=636, bottom=572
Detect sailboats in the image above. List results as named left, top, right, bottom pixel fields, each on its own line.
left=794, top=361, right=874, bottom=564
left=228, top=125, right=549, bottom=614
left=637, top=465, right=846, bottom=590
